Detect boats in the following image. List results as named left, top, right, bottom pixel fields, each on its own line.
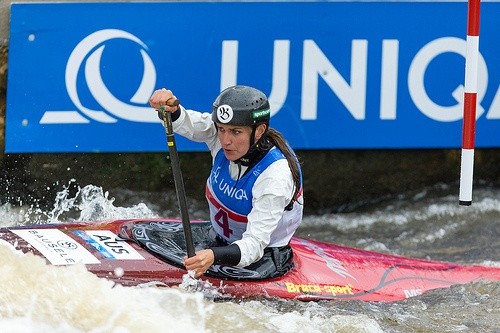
left=0, top=218, right=500, bottom=306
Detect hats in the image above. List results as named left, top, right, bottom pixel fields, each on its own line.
left=212, top=85, right=271, bottom=124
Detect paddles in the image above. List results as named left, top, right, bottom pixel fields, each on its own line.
left=162, top=98, right=202, bottom=280
